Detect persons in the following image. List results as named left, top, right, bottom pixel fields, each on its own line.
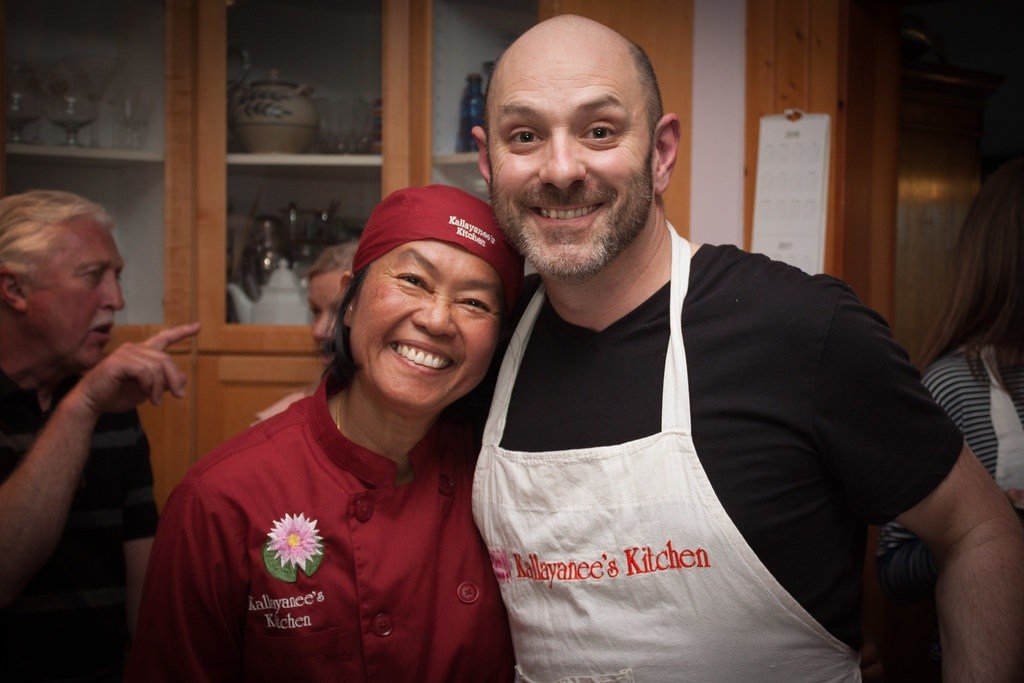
left=131, top=14, right=1024, bottom=683
left=0, top=190, right=200, bottom=683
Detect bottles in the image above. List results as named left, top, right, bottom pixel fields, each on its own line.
left=462, top=74, right=484, bottom=152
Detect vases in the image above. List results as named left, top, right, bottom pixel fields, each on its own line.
left=462, top=73, right=485, bottom=152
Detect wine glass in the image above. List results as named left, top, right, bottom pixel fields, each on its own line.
left=44, top=63, right=96, bottom=148
left=113, top=86, right=151, bottom=150
left=65, top=55, right=126, bottom=149
left=6, top=61, right=43, bottom=145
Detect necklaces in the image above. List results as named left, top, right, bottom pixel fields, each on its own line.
left=337, top=390, right=342, bottom=431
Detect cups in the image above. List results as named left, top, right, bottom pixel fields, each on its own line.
left=318, top=100, right=374, bottom=152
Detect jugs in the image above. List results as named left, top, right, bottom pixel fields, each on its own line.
left=227, top=258, right=315, bottom=326
left=230, top=70, right=317, bottom=156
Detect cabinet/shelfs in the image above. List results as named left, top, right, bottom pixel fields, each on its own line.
left=0, top=0, right=585, bottom=512
